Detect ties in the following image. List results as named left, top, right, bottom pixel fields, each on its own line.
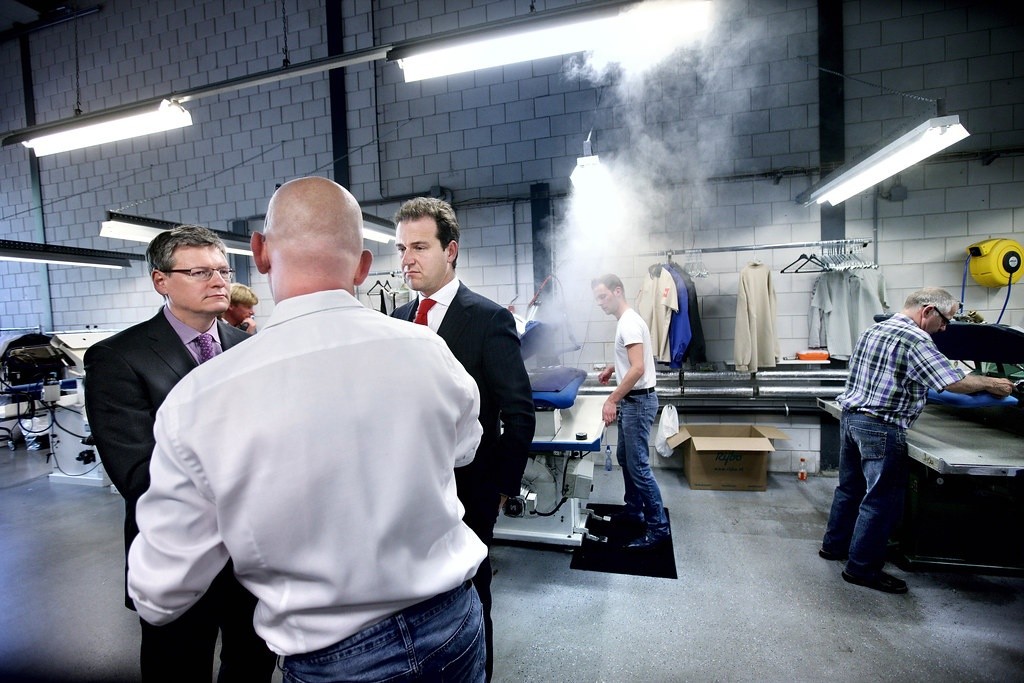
left=414, top=299, right=437, bottom=326
left=198, top=333, right=215, bottom=364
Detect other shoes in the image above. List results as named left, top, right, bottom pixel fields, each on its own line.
left=842, top=568, right=908, bottom=594
left=623, top=525, right=671, bottom=552
left=819, top=548, right=849, bottom=561
left=606, top=508, right=645, bottom=523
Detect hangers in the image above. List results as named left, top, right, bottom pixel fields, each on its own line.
left=655, top=250, right=709, bottom=278
left=749, top=246, right=762, bottom=264
left=780, top=238, right=880, bottom=273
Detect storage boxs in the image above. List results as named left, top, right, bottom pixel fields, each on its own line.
left=671, top=424, right=790, bottom=492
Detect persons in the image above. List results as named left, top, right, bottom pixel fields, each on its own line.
left=591, top=275, right=669, bottom=549
left=819, top=289, right=1014, bottom=594
left=129, top=176, right=496, bottom=683
left=387, top=197, right=536, bottom=683
left=84, top=226, right=279, bottom=683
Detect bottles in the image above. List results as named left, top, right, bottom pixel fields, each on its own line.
left=605, top=445, right=612, bottom=471
left=798, top=458, right=807, bottom=485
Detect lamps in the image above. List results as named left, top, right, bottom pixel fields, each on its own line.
left=386, top=1, right=715, bottom=82
left=274, top=183, right=396, bottom=243
left=797, top=116, right=972, bottom=208
left=99, top=210, right=253, bottom=258
left=0, top=249, right=133, bottom=270
left=20, top=99, right=192, bottom=157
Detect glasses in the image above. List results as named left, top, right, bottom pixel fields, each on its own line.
left=160, top=266, right=235, bottom=279
left=923, top=304, right=950, bottom=325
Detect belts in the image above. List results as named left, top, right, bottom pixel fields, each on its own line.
left=623, top=387, right=654, bottom=403
